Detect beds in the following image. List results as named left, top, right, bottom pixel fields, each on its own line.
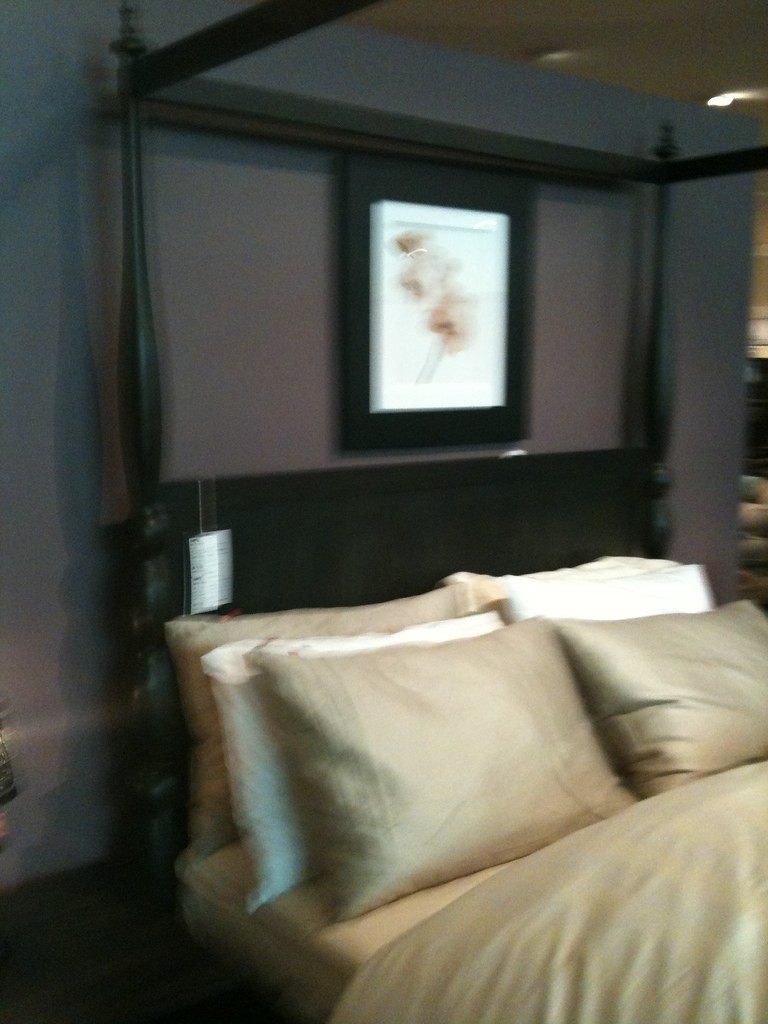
left=171, top=551, right=768, bottom=1024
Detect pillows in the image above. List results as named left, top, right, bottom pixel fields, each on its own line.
left=559, top=595, right=768, bottom=795
left=246, top=615, right=636, bottom=916
left=163, top=584, right=488, bottom=867
left=440, top=553, right=682, bottom=627
left=197, top=606, right=503, bottom=913
left=503, top=562, right=717, bottom=634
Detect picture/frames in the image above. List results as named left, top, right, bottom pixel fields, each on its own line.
left=338, top=147, right=539, bottom=452
